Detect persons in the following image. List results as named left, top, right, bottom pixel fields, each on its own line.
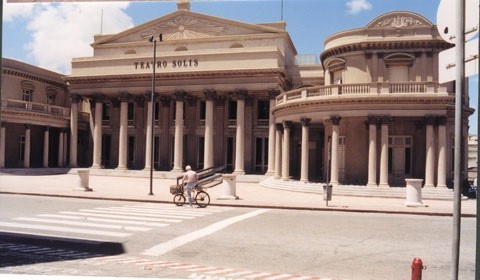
left=186, top=166, right=199, bottom=207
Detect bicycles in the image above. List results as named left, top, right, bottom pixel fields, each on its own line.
left=170, top=183, right=210, bottom=208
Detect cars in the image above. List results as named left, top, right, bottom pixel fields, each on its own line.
left=468, top=185, right=477, bottom=198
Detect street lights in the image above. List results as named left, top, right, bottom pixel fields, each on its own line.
left=147, top=32, right=164, bottom=195
